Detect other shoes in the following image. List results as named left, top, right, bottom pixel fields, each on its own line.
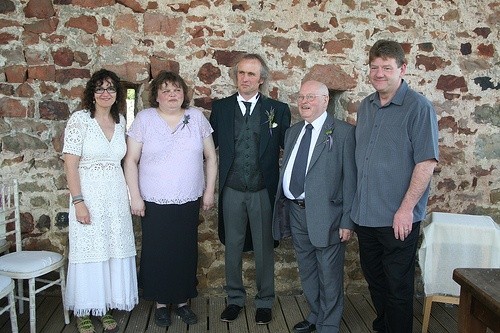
left=176, top=305, right=198, bottom=324
left=154, top=305, right=171, bottom=327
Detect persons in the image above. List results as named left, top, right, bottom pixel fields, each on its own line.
left=271, top=80, right=357, bottom=333
left=61, top=69, right=139, bottom=333
left=124, top=71, right=218, bottom=327
left=203, top=54, right=291, bottom=325
left=349, top=40, right=439, bottom=333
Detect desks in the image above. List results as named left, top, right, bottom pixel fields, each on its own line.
left=417, top=212, right=500, bottom=333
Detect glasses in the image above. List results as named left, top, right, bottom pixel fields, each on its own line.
left=297, top=94, right=328, bottom=103
left=95, top=86, right=117, bottom=94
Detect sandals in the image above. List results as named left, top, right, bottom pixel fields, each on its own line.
left=97, top=314, right=119, bottom=333
left=77, top=315, right=96, bottom=333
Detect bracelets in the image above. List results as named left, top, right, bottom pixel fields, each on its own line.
left=72, top=195, right=84, bottom=205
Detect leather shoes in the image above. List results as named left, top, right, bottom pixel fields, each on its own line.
left=294, top=320, right=316, bottom=333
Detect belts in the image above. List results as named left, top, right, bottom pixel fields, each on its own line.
left=291, top=199, right=305, bottom=207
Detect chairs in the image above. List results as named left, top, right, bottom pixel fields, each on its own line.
left=0, top=178, right=70, bottom=333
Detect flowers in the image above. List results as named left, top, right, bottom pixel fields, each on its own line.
left=263, top=105, right=279, bottom=135
left=323, top=122, right=334, bottom=150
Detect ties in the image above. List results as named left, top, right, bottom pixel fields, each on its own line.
left=242, top=101, right=252, bottom=124
left=289, top=124, right=314, bottom=199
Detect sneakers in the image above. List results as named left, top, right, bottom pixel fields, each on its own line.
left=220, top=304, right=244, bottom=322
left=255, top=308, right=273, bottom=324
